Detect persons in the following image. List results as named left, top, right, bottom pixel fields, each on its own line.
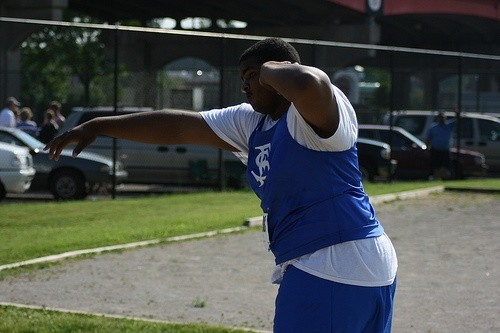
left=43, top=38, right=398, bottom=333
left=0, top=96, right=65, bottom=147
left=425, top=103, right=460, bottom=181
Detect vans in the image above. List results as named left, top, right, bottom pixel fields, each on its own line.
left=383, top=110, right=500, bottom=179
left=55, top=108, right=250, bottom=190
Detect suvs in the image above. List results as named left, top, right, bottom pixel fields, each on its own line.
left=357, top=111, right=488, bottom=183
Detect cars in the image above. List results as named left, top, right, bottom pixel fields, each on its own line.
left=0, top=126, right=128, bottom=202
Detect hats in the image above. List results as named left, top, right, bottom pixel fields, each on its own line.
left=7, top=97, right=21, bottom=106
